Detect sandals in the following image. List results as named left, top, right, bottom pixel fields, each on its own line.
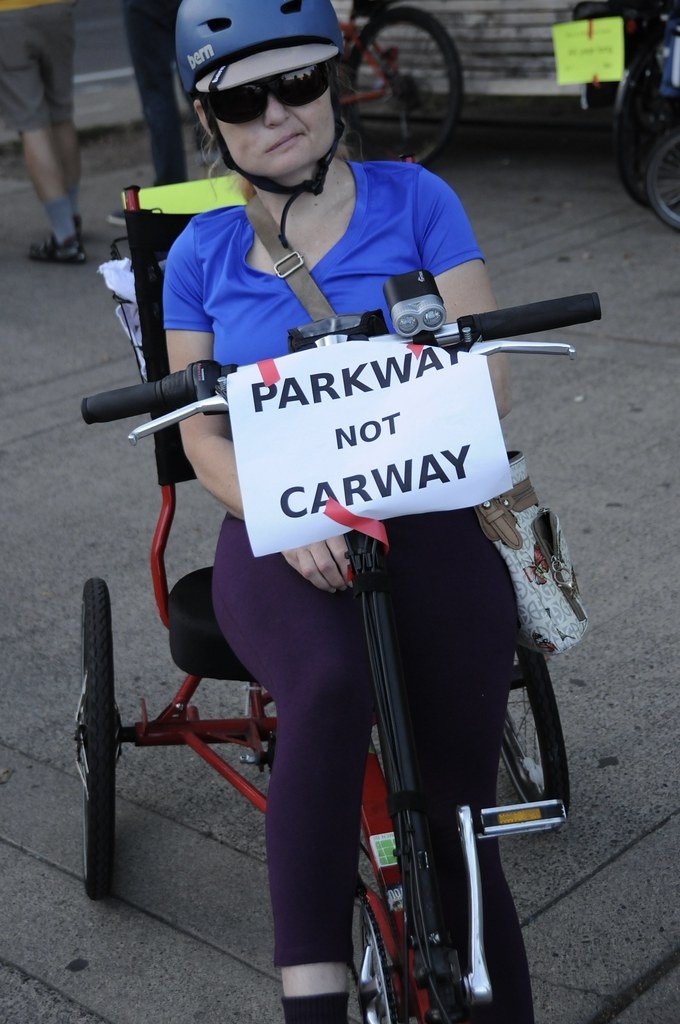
left=29, top=214, right=87, bottom=263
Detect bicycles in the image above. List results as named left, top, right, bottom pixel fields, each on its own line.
left=322, top=1, right=467, bottom=170
left=569, top=0, right=680, bottom=229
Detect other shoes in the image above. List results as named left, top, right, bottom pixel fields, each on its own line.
left=281, top=989, right=347, bottom=1024
left=108, top=207, right=126, bottom=228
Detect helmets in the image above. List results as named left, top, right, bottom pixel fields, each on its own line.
left=175, top=0, right=342, bottom=104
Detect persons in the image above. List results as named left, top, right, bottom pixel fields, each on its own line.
left=0, top=0, right=187, bottom=263
left=161, top=0, right=538, bottom=1024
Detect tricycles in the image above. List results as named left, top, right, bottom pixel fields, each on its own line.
left=70, top=192, right=605, bottom=1024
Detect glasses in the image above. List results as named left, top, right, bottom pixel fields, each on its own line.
left=199, top=60, right=334, bottom=124
